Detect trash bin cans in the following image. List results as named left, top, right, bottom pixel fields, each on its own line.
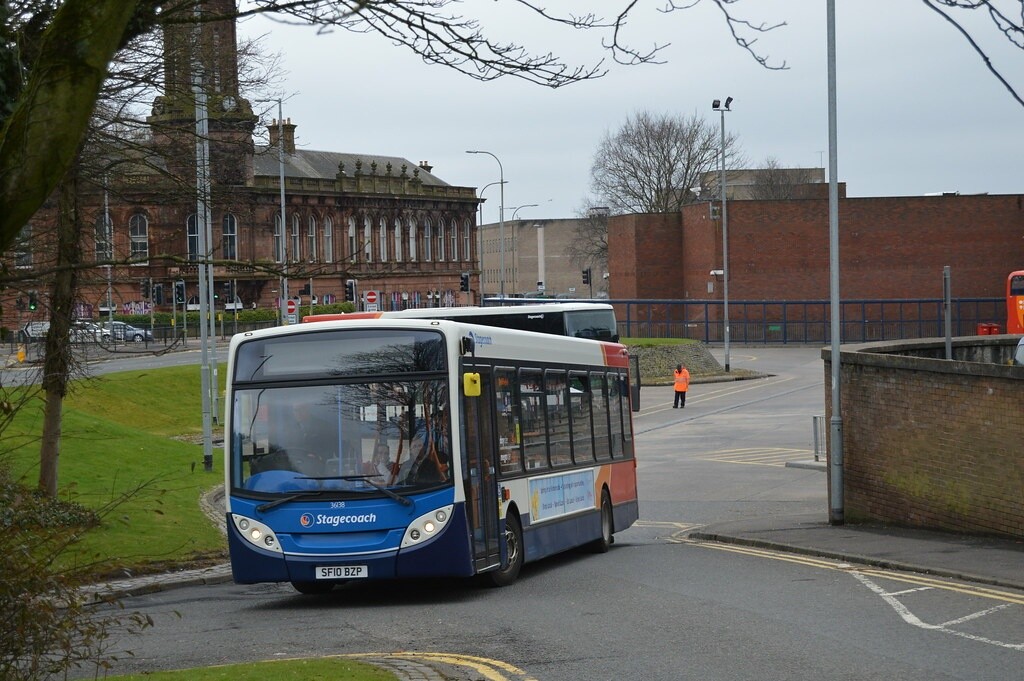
left=976, top=323, right=992, bottom=336
left=988, top=322, right=1001, bottom=336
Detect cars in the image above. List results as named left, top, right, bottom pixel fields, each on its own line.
left=69, top=322, right=111, bottom=343
left=97, top=321, right=153, bottom=342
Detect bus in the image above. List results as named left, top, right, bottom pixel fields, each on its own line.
left=222, top=317, right=640, bottom=593
left=302, top=302, right=619, bottom=346
left=1007, top=269, right=1024, bottom=334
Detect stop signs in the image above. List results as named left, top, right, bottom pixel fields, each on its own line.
left=366, top=291, right=378, bottom=303
left=288, top=301, right=296, bottom=314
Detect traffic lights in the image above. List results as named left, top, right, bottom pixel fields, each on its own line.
left=711, top=96, right=734, bottom=372
left=154, top=284, right=164, bottom=307
left=345, top=280, right=356, bottom=303
left=176, top=281, right=186, bottom=304
left=459, top=273, right=470, bottom=294
left=15, top=297, right=22, bottom=310
left=140, top=280, right=149, bottom=300
left=581, top=267, right=590, bottom=285
left=29, top=293, right=38, bottom=311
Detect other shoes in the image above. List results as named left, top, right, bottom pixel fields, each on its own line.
left=673, top=405, right=678, bottom=408
left=681, top=405, right=684, bottom=408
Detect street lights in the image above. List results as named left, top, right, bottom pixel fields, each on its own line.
left=465, top=150, right=505, bottom=296
left=511, top=203, right=539, bottom=299
left=479, top=180, right=509, bottom=307
left=254, top=97, right=289, bottom=325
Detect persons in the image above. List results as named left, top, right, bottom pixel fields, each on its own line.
left=431, top=410, right=447, bottom=430
left=672, top=364, right=690, bottom=409
left=280, top=398, right=333, bottom=471
left=392, top=434, right=439, bottom=484
left=371, top=443, right=399, bottom=484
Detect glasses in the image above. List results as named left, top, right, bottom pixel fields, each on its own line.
left=411, top=444, right=422, bottom=450
